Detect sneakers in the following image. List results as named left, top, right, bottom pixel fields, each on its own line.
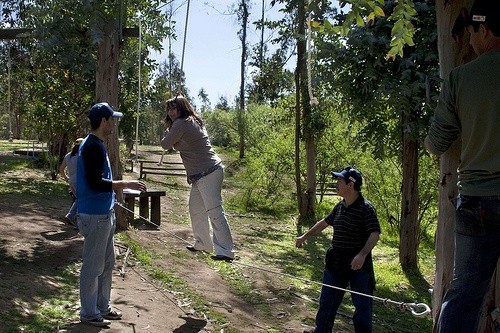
left=101, top=310, right=122, bottom=320
left=79, top=315, right=111, bottom=326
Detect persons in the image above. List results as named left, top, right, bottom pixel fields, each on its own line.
left=296, top=169, right=381, bottom=333
left=61, top=138, right=84, bottom=226
left=161, top=98, right=234, bottom=260
left=75, top=102, right=146, bottom=326
left=424, top=0, right=500, bottom=333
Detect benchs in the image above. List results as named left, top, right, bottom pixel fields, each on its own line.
left=136, top=159, right=187, bottom=181
left=290, top=180, right=337, bottom=202
left=121, top=187, right=166, bottom=229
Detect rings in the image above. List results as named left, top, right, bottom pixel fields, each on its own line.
left=358, top=265, right=360, bottom=267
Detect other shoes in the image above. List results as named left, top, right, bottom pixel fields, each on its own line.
left=65, top=217, right=74, bottom=226
left=211, top=254, right=232, bottom=263
left=186, top=245, right=209, bottom=253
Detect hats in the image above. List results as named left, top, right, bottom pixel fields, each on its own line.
left=89, top=103, right=123, bottom=119
left=75, top=138, right=83, bottom=145
left=469, top=0, right=500, bottom=22
left=331, top=169, right=362, bottom=186
